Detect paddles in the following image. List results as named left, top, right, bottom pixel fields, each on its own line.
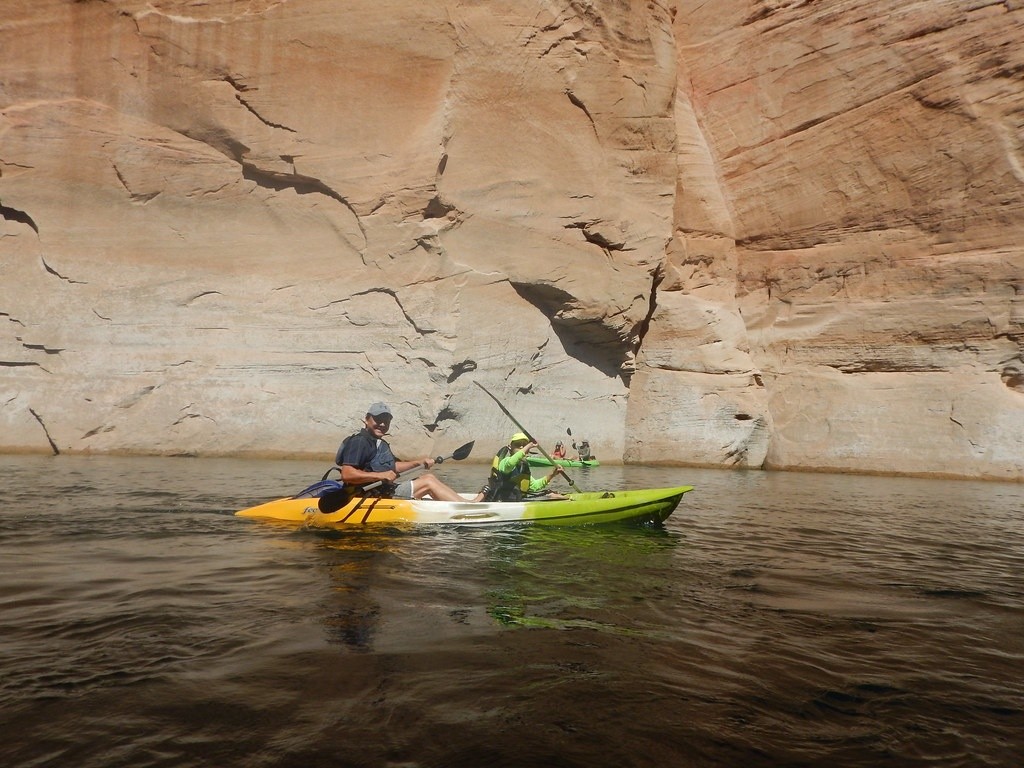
left=566, top=428, right=589, bottom=462
left=472, top=379, right=584, bottom=494
left=318, top=439, right=477, bottom=514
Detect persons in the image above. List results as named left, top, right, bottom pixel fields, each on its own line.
left=341, top=401, right=490, bottom=502
left=491, top=433, right=570, bottom=500
left=572, top=439, right=590, bottom=461
left=551, top=440, right=566, bottom=460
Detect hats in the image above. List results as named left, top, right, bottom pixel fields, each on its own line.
left=367, top=402, right=393, bottom=420
left=582, top=439, right=588, bottom=442
left=556, top=441, right=562, bottom=445
left=510, top=433, right=529, bottom=445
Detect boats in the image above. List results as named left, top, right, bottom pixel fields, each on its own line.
left=525, top=456, right=600, bottom=469
left=232, top=483, right=694, bottom=534
